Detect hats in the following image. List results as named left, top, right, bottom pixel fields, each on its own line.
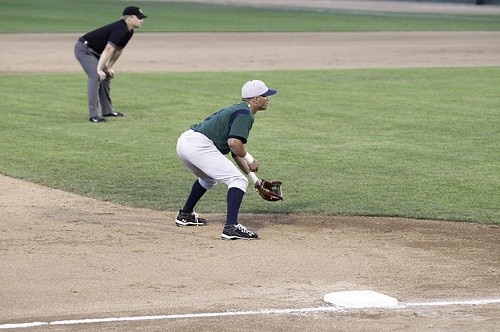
left=123, top=6, right=146, bottom=18
left=242, top=80, right=276, bottom=98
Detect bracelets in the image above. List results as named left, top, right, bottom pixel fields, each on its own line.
left=242, top=151, right=255, bottom=164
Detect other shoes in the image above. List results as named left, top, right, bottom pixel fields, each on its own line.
left=89, top=116, right=105, bottom=122
left=102, top=112, right=123, bottom=116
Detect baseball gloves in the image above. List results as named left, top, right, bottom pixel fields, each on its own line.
left=256, top=178, right=284, bottom=203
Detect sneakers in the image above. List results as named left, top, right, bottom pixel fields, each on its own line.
left=221, top=223, right=257, bottom=240
left=174, top=209, right=206, bottom=225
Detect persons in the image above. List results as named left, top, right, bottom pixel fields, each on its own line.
left=73, top=5, right=147, bottom=121
left=173, top=80, right=278, bottom=240
